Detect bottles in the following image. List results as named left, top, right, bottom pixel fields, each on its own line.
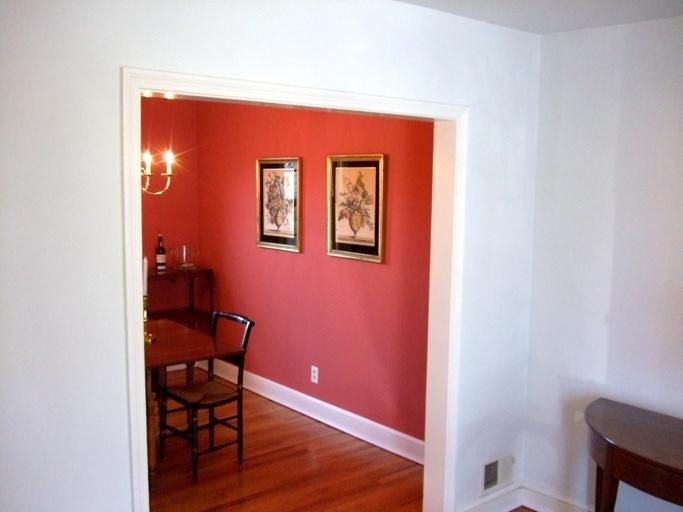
left=155, top=233, right=167, bottom=271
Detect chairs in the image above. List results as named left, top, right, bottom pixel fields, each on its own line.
left=156, top=311, right=254, bottom=477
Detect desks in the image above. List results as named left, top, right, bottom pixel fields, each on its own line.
left=143, top=318, right=246, bottom=474
left=581, top=395, right=682, bottom=512
left=141, top=266, right=215, bottom=381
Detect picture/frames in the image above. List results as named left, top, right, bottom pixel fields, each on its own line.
left=326, top=154, right=385, bottom=265
left=255, top=156, right=301, bottom=254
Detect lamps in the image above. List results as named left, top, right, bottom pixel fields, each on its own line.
left=140, top=148, right=173, bottom=196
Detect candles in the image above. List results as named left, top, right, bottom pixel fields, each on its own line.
left=142, top=255, right=148, bottom=296
